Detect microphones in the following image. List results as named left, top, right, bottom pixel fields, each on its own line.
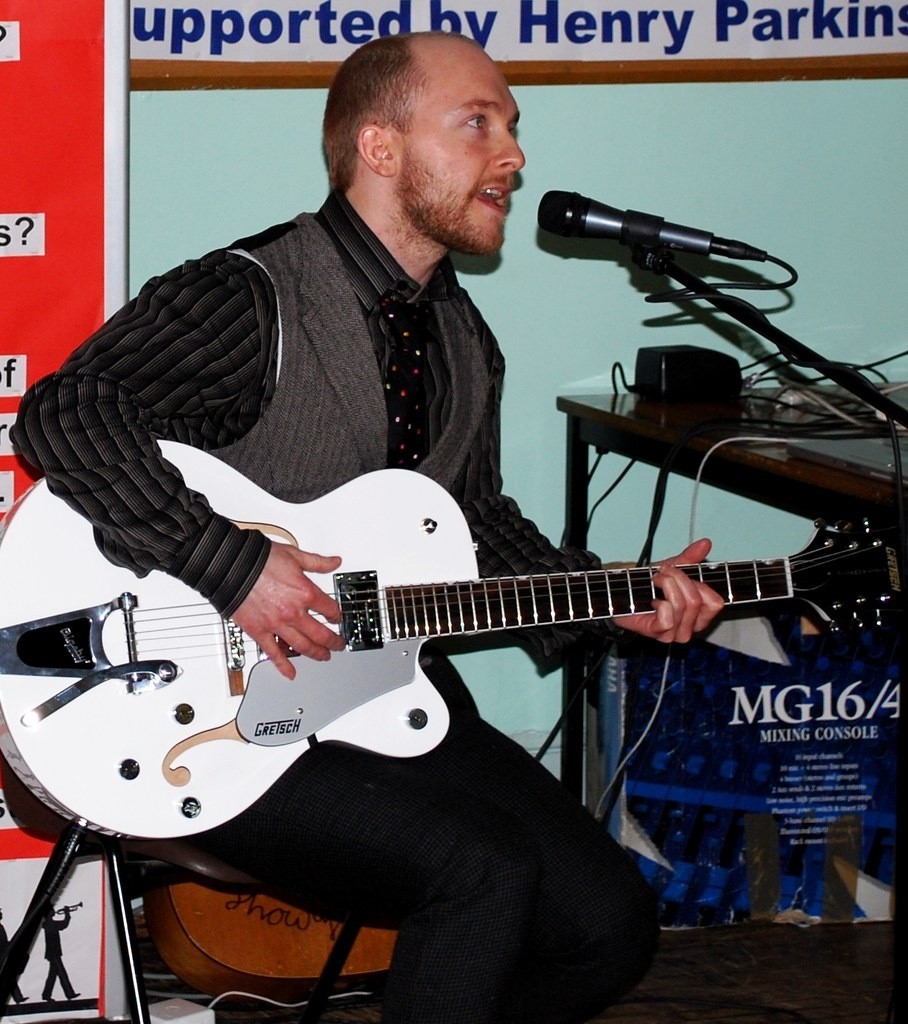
left=536, top=192, right=770, bottom=264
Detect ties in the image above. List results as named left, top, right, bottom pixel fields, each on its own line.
left=378, top=292, right=429, bottom=474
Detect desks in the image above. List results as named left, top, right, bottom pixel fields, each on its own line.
left=547, top=383, right=907, bottom=867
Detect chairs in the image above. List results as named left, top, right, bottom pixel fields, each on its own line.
left=2, top=805, right=368, bottom=1024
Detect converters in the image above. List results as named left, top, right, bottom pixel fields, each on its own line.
left=634, top=344, right=742, bottom=404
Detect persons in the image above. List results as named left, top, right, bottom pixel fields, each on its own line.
left=11, top=34, right=729, bottom=1024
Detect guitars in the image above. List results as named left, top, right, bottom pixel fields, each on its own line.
left=142, top=883, right=400, bottom=1003
left=0, top=440, right=908, bottom=839
